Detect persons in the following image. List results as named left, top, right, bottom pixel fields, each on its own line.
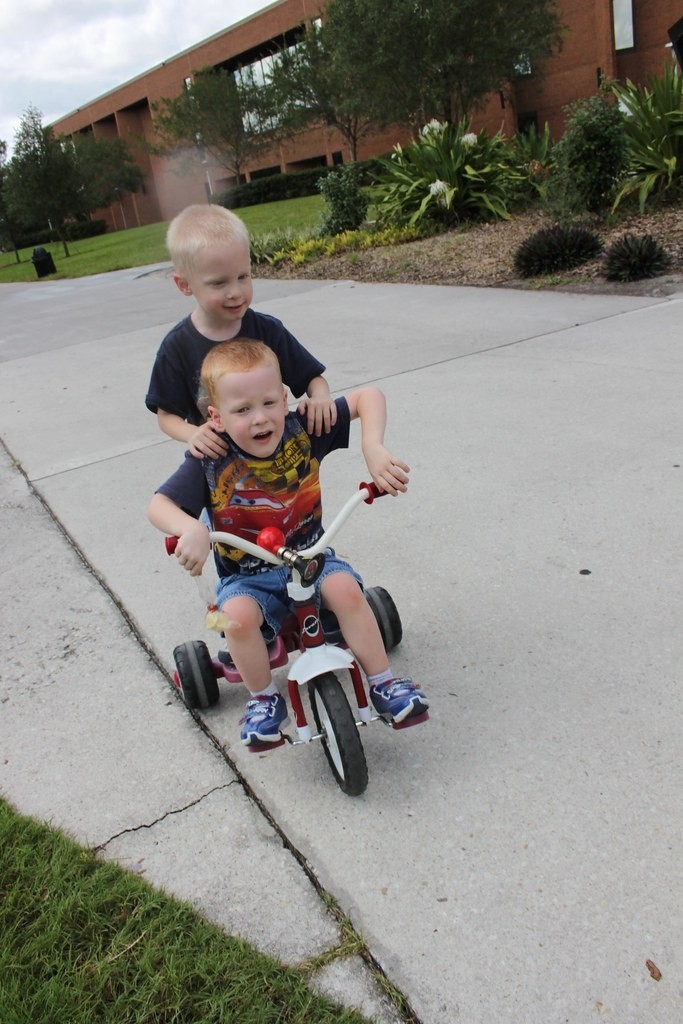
left=147, top=338, right=432, bottom=747
left=146, top=203, right=344, bottom=665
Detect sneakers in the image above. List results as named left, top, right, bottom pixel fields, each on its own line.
left=238, top=691, right=292, bottom=746
left=369, top=675, right=429, bottom=724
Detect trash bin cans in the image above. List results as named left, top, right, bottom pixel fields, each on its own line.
left=30, top=247, right=57, bottom=278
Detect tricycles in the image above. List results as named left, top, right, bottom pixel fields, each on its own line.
left=160, top=476, right=434, bottom=797
